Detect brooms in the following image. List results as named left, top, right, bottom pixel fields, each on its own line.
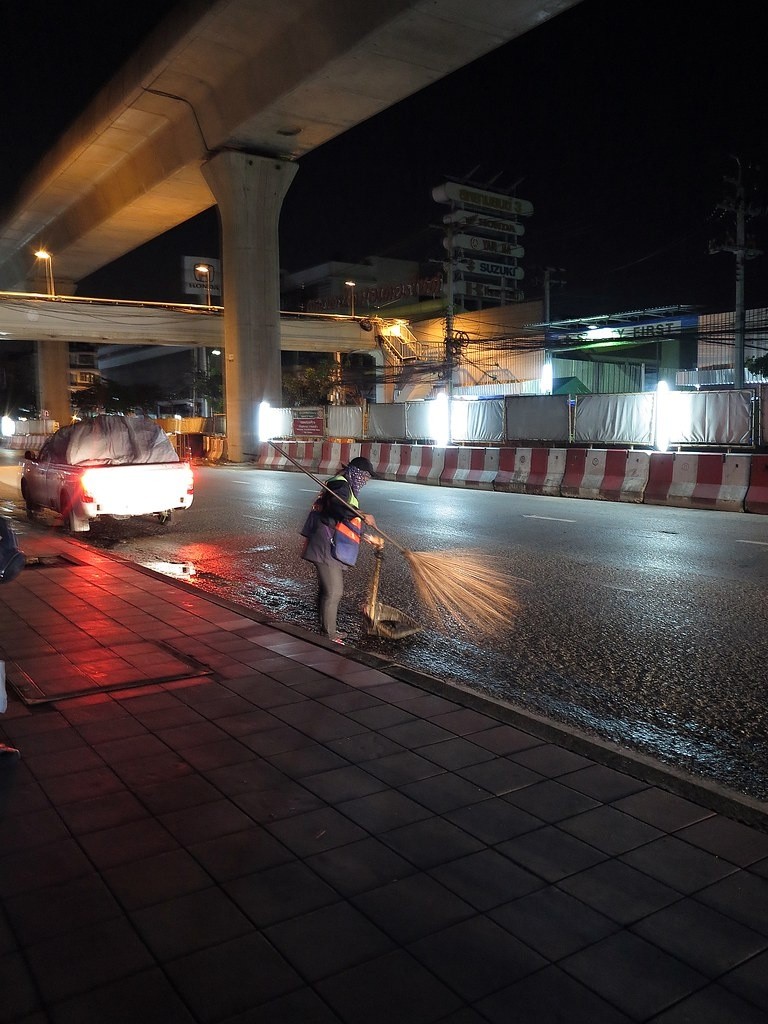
left=266, top=438, right=535, bottom=639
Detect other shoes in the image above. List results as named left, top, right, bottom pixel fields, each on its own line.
left=332, top=638, right=346, bottom=646
left=0, top=745, right=20, bottom=766
left=339, top=632, right=348, bottom=639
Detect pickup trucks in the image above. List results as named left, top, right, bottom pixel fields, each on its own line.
left=19, top=412, right=195, bottom=538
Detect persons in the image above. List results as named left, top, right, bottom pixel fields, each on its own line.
left=299, top=456, right=378, bottom=647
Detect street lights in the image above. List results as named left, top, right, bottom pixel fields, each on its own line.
left=345, top=281, right=355, bottom=317
left=34, top=250, right=56, bottom=295
left=196, top=266, right=211, bottom=306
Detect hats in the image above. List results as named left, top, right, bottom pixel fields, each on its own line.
left=348, top=456, right=376, bottom=476
